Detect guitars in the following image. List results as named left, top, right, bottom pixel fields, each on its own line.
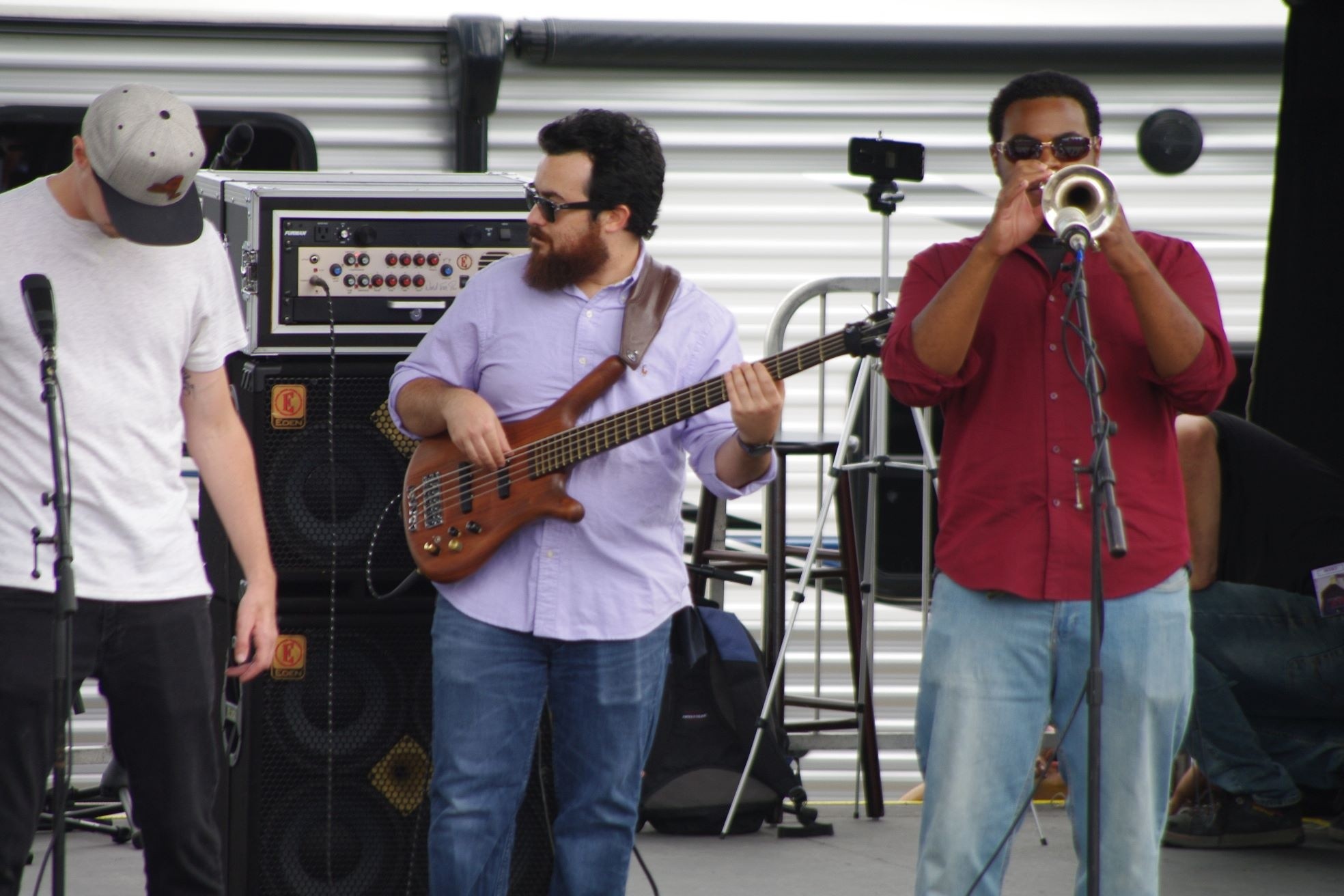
left=401, top=306, right=898, bottom=584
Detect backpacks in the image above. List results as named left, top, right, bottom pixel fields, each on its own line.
left=641, top=599, right=784, bottom=834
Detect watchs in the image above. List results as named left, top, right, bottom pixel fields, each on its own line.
left=736, top=430, right=776, bottom=459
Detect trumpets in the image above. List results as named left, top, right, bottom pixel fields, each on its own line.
left=1038, top=164, right=1119, bottom=239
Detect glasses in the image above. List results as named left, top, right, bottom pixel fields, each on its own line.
left=525, top=181, right=614, bottom=224
left=995, top=135, right=1100, bottom=162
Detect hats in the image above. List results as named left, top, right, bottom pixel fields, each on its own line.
left=80, top=83, right=208, bottom=247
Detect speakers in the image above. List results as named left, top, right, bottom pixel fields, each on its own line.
left=195, top=349, right=559, bottom=895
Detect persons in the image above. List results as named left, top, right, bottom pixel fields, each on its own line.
left=0, top=82, right=284, bottom=896
left=387, top=105, right=783, bottom=896
left=1162, top=405, right=1344, bottom=853
left=883, top=72, right=1237, bottom=896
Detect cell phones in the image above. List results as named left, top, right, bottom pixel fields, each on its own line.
left=848, top=138, right=925, bottom=183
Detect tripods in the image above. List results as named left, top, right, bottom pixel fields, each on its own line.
left=719, top=180, right=1048, bottom=846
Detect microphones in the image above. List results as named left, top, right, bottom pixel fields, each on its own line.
left=211, top=123, right=255, bottom=171
left=20, top=273, right=57, bottom=376
left=1056, top=205, right=1092, bottom=256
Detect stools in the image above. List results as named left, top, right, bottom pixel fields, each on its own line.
left=688, top=431, right=887, bottom=821
